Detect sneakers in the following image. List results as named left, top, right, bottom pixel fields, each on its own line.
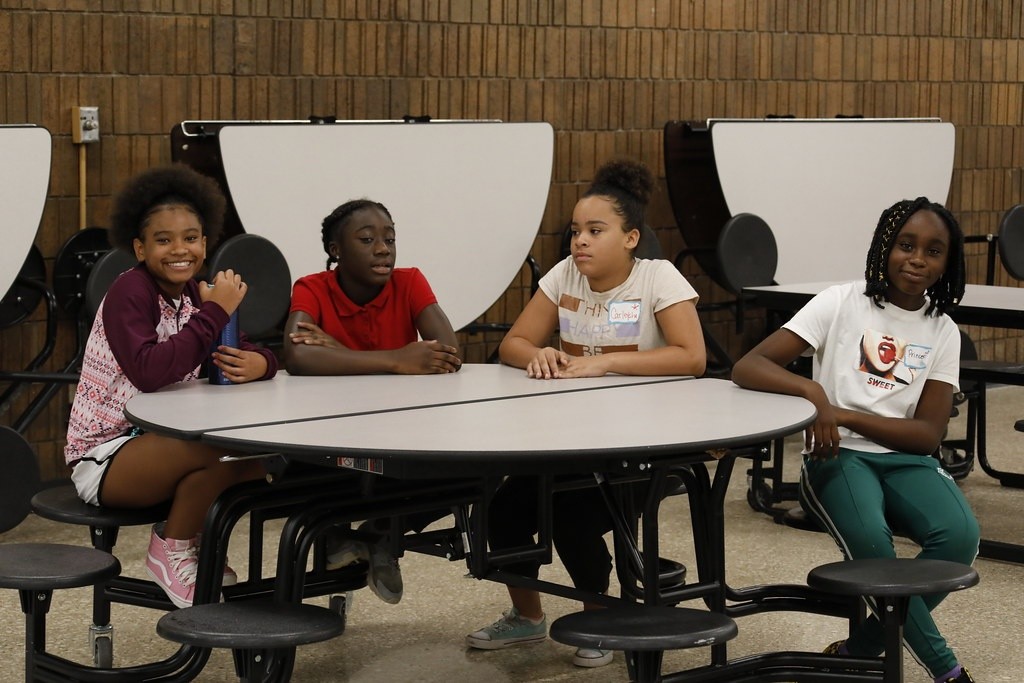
left=465, top=606, right=548, bottom=651
left=324, top=523, right=403, bottom=605
left=572, top=646, right=614, bottom=667
left=144, top=522, right=238, bottom=608
left=943, top=667, right=975, bottom=683
left=822, top=639, right=846, bottom=656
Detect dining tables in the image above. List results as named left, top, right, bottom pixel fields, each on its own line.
left=118, top=350, right=822, bottom=683
left=702, top=115, right=958, bottom=307
left=741, top=272, right=1024, bottom=469
left=168, top=113, right=560, bottom=354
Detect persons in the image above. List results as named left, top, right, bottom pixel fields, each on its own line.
left=466, top=155, right=706, bottom=668
left=64, top=166, right=279, bottom=610
left=284, top=199, right=460, bottom=605
left=731, top=191, right=983, bottom=683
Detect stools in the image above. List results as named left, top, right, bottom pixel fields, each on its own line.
left=31, top=484, right=185, bottom=671
left=998, top=204, right=1024, bottom=282
left=158, top=590, right=350, bottom=683
left=1, top=423, right=43, bottom=538
left=718, top=211, right=781, bottom=297
left=54, top=226, right=139, bottom=321
left=804, top=554, right=981, bottom=680
left=546, top=599, right=739, bottom=683
left=0, top=538, right=118, bottom=683
